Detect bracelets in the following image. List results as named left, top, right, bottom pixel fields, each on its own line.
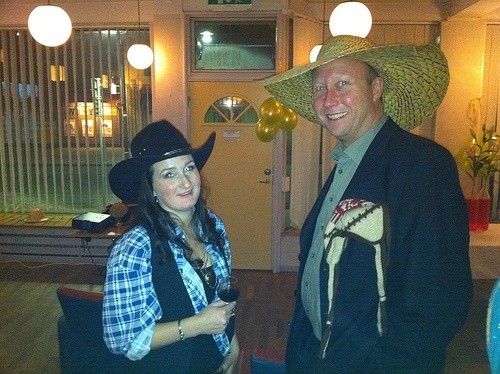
left=178, top=319, right=185, bottom=341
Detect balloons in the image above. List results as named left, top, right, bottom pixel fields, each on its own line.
left=259, top=97, right=283, bottom=123
left=278, top=105, right=298, bottom=131
left=256, top=119, right=277, bottom=142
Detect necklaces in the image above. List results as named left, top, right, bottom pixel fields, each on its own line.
left=190, top=251, right=212, bottom=285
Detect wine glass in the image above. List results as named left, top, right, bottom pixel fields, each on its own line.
left=217, top=276, right=240, bottom=305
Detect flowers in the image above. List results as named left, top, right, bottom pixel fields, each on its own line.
left=461, top=122, right=500, bottom=197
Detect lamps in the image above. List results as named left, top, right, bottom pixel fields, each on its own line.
left=329, top=0, right=373, bottom=38
left=127, top=0, right=153, bottom=69
left=200, top=29, right=214, bottom=44
left=28, top=0, right=72, bottom=47
left=309, top=0, right=326, bottom=63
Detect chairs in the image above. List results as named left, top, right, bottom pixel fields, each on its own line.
left=56, top=286, right=126, bottom=374
left=239, top=347, right=285, bottom=374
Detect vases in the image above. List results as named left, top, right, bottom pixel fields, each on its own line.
left=467, top=190, right=490, bottom=232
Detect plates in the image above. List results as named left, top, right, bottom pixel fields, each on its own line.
left=24, top=218, right=49, bottom=222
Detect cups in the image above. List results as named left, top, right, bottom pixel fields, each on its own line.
left=28, top=208, right=43, bottom=220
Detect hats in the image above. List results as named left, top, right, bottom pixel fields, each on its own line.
left=108, top=121, right=217, bottom=209
left=252, top=34, right=449, bottom=130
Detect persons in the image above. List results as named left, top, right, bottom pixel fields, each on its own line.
left=102, top=119, right=240, bottom=374
left=256, top=35, right=473, bottom=374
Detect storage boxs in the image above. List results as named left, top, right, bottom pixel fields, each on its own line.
left=72, top=212, right=113, bottom=234
